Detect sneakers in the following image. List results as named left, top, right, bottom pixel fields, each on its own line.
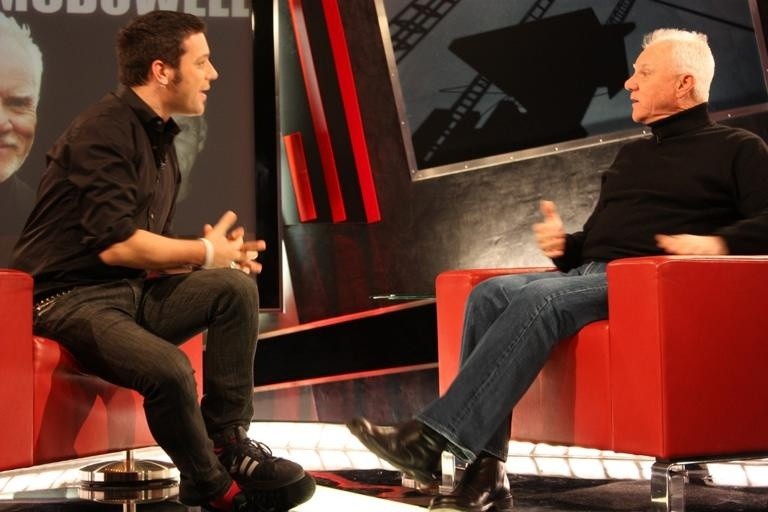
left=201, top=424, right=315, bottom=511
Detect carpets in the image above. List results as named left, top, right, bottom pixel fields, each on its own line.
left=305, top=469, right=768, bottom=512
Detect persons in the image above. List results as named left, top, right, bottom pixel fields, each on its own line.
left=164, top=114, right=218, bottom=238
left=344, top=28, right=768, bottom=511
left=7, top=10, right=316, bottom=512
left=0, top=11, right=44, bottom=184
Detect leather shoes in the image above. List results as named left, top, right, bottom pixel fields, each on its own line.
left=427, top=456, right=515, bottom=512
left=347, top=417, right=444, bottom=486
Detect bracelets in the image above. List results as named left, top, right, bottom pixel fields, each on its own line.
left=199, top=236, right=214, bottom=271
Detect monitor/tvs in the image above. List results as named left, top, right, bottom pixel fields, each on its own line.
left=0, top=0, right=284, bottom=313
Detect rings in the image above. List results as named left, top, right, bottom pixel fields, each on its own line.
left=229, top=259, right=236, bottom=270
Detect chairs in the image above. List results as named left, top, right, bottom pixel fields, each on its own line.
left=0, top=269, right=203, bottom=490
left=435, top=255, right=768, bottom=512
